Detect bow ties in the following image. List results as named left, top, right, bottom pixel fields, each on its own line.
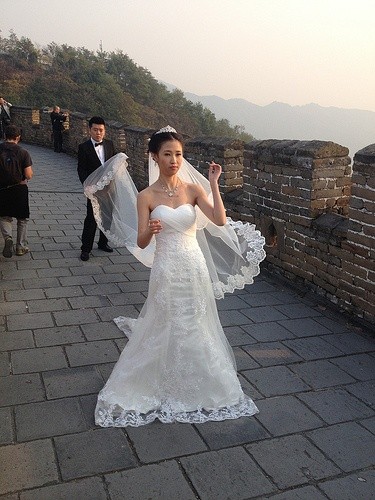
left=94, top=142, right=104, bottom=147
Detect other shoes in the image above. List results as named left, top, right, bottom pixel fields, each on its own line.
left=2, top=238, right=13, bottom=258
left=15, top=246, right=33, bottom=255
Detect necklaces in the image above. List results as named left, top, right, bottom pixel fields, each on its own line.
left=158, top=177, right=180, bottom=198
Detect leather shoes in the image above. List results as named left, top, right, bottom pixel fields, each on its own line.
left=80, top=250, right=89, bottom=261
left=98, top=243, right=114, bottom=252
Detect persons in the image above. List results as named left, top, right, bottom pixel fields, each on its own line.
left=76, top=117, right=129, bottom=262
left=0, top=126, right=33, bottom=258
left=136, top=125, right=228, bottom=383
left=50, top=105, right=67, bottom=154
left=0, top=97, right=13, bottom=139
